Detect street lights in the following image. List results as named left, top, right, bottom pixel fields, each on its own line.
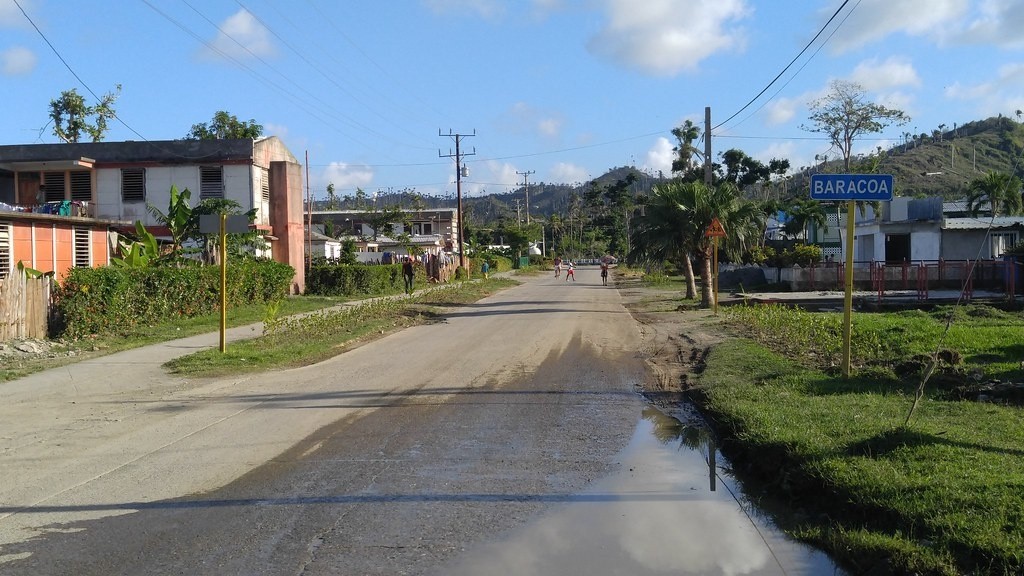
left=526, top=206, right=539, bottom=225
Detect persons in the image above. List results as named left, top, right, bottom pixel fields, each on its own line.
left=35, top=185, right=45, bottom=206
left=554, top=255, right=561, bottom=278
left=481, top=260, right=489, bottom=279
left=825, top=255, right=829, bottom=268
left=566, top=258, right=576, bottom=282
left=600, top=257, right=609, bottom=286
left=402, top=257, right=415, bottom=293
left=828, top=254, right=835, bottom=268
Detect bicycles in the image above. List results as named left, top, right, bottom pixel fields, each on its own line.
left=554, top=266, right=561, bottom=278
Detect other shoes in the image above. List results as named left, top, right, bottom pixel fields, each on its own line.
left=603, top=284, right=604, bottom=286
left=605, top=284, right=607, bottom=286
left=566, top=280, right=569, bottom=281
left=573, top=279, right=575, bottom=281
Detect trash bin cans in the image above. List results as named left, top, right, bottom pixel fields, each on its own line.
left=513, top=256, right=529, bottom=269
